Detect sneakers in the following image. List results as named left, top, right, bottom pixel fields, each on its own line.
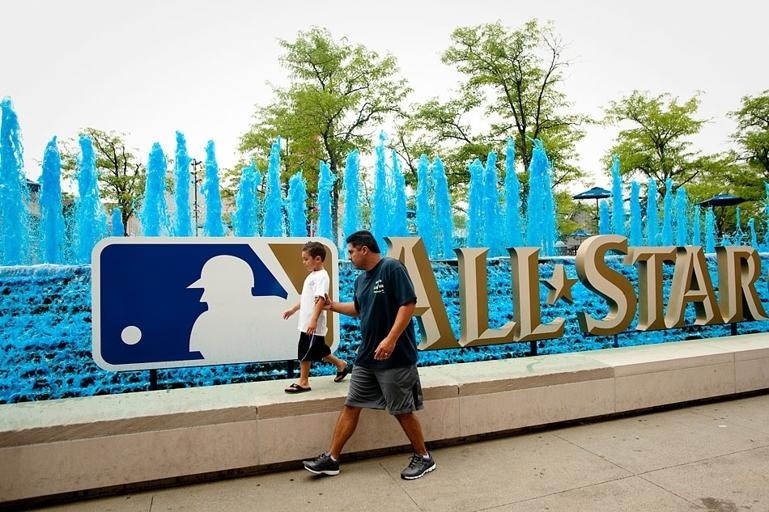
left=302, top=452, right=340, bottom=476
left=401, top=451, right=436, bottom=481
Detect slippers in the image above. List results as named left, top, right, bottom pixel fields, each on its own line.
left=285, top=383, right=312, bottom=394
left=333, top=364, right=353, bottom=382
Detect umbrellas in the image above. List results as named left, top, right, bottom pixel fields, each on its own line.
left=572, top=185, right=616, bottom=234
left=698, top=190, right=749, bottom=230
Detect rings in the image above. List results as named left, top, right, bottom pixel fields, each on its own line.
left=385, top=353, right=389, bottom=355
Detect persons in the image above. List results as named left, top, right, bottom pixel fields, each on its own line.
left=302, top=228, right=438, bottom=480
left=281, top=241, right=353, bottom=393
